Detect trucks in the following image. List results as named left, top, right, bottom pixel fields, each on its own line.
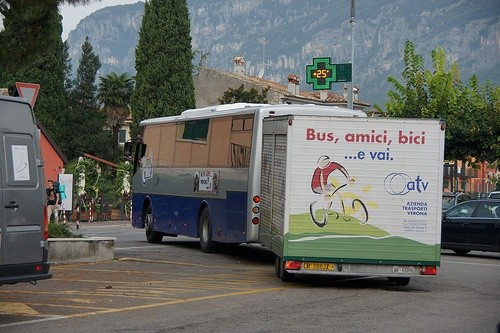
left=0, top=95, right=54, bottom=287
left=258, top=115, right=446, bottom=287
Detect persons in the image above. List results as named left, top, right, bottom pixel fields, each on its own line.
left=45, top=179, right=59, bottom=226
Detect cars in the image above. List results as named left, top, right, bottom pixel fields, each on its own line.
left=441, top=200, right=500, bottom=256
left=487, top=191, right=500, bottom=200
left=441, top=192, right=472, bottom=223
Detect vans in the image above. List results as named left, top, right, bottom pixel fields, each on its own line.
left=123, top=102, right=368, bottom=253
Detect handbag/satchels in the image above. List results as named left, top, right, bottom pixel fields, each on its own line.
left=48, top=200, right=56, bottom=205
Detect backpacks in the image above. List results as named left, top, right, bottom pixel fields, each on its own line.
left=47, top=182, right=62, bottom=204
